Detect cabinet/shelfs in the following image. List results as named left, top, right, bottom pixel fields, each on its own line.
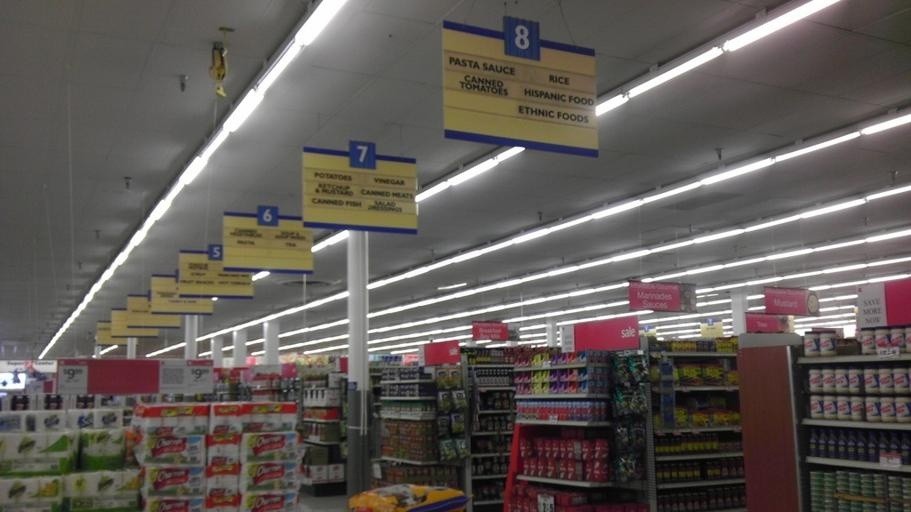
left=368, top=362, right=380, bottom=484
left=796, top=330, right=911, bottom=510
left=381, top=366, right=439, bottom=486
left=303, top=382, right=348, bottom=496
left=464, top=349, right=516, bottom=509
left=514, top=348, right=612, bottom=510
left=648, top=337, right=747, bottom=511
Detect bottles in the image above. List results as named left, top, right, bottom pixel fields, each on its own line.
left=464, top=353, right=511, bottom=507
left=798, top=324, right=911, bottom=464
left=511, top=399, right=611, bottom=421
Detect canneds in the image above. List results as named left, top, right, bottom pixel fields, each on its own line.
left=654, top=433, right=747, bottom=512
left=804, top=325, right=911, bottom=422
